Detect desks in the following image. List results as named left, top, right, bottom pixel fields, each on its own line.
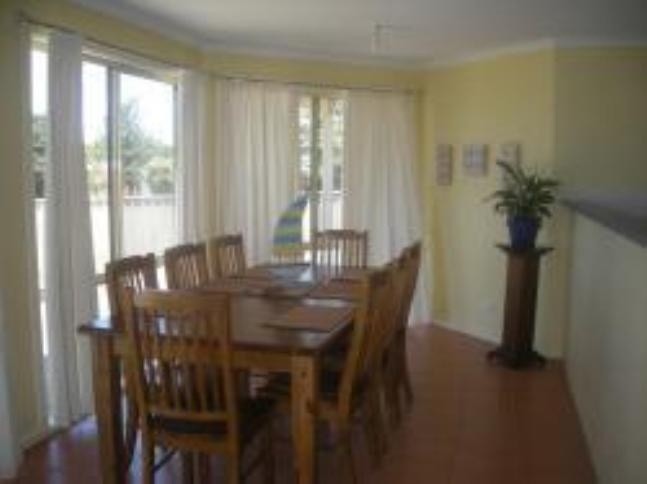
left=484, top=242, right=555, bottom=373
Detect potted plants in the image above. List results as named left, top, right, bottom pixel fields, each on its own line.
left=480, top=141, right=569, bottom=247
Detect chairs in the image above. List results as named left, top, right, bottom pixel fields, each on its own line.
left=76, top=227, right=423, bottom=484
